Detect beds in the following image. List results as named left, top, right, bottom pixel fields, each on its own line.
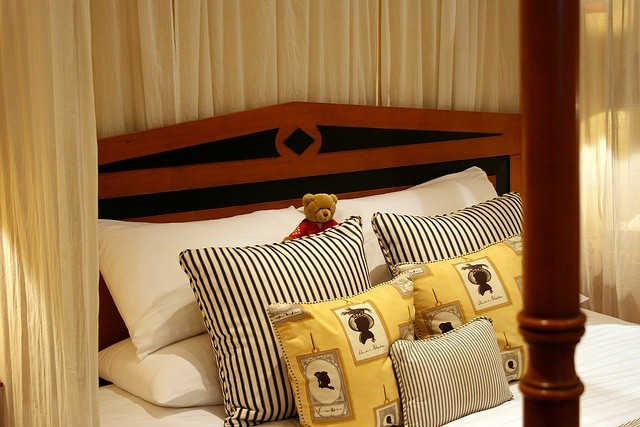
left=97, top=102, right=640, bottom=427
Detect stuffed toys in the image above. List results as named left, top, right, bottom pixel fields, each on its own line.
left=284, top=193, right=339, bottom=242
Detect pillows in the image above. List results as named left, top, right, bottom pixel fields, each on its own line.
left=298, top=166, right=500, bottom=285
left=395, top=234, right=533, bottom=382
left=268, top=272, right=417, bottom=427
left=372, top=190, right=524, bottom=277
left=388, top=315, right=514, bottom=427
left=97, top=206, right=304, bottom=358
left=179, top=215, right=371, bottom=427
left=97, top=333, right=223, bottom=407
